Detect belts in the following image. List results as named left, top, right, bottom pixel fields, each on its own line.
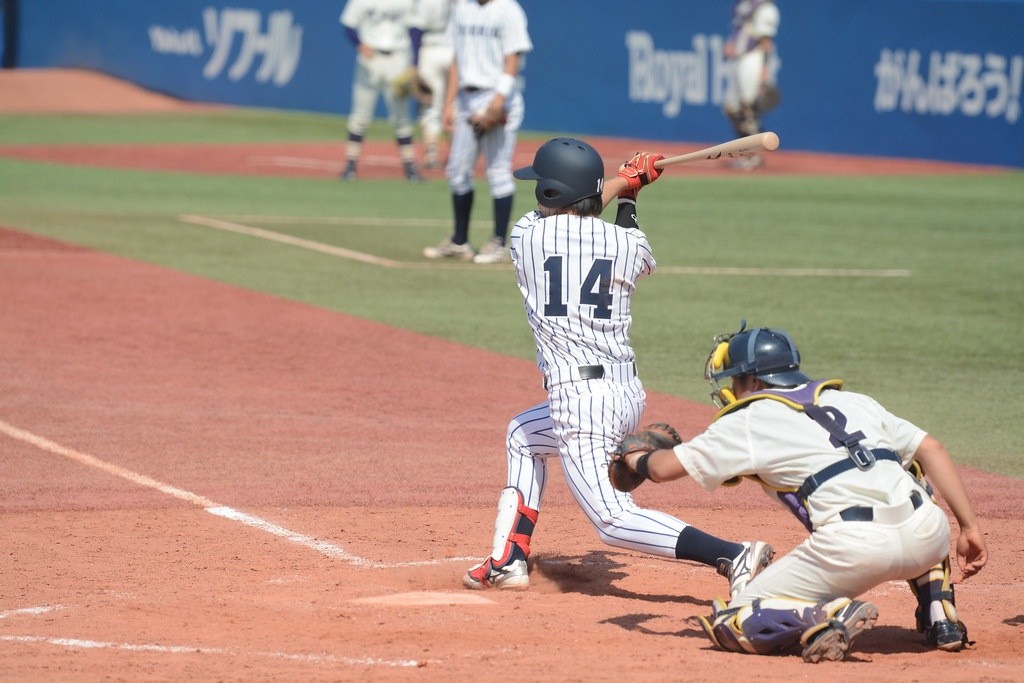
left=578, top=363, right=637, bottom=380
left=839, top=491, right=924, bottom=521
left=467, top=86, right=476, bottom=91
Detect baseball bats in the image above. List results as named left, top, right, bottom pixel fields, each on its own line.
left=655, top=130, right=780, bottom=169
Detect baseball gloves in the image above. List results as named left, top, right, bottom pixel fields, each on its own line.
left=608, top=425, right=683, bottom=492
left=469, top=107, right=504, bottom=135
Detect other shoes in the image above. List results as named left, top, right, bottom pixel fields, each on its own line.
left=423, top=238, right=470, bottom=258
left=406, top=164, right=420, bottom=178
left=343, top=163, right=355, bottom=179
left=473, top=246, right=508, bottom=264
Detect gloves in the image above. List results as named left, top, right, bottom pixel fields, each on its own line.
left=617, top=153, right=664, bottom=201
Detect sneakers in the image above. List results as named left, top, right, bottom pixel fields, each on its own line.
left=463, top=559, right=529, bottom=589
left=717, top=541, right=776, bottom=599
left=802, top=601, right=878, bottom=663
left=925, top=618, right=961, bottom=649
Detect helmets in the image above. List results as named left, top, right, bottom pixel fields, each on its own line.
left=729, top=328, right=808, bottom=385
left=513, top=137, right=603, bottom=208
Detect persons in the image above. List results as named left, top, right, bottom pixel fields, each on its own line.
left=723, top=0, right=780, bottom=170
left=464, top=137, right=773, bottom=594
left=416, top=0, right=533, bottom=264
left=339, top=0, right=423, bottom=185
left=627, top=328, right=988, bottom=661
